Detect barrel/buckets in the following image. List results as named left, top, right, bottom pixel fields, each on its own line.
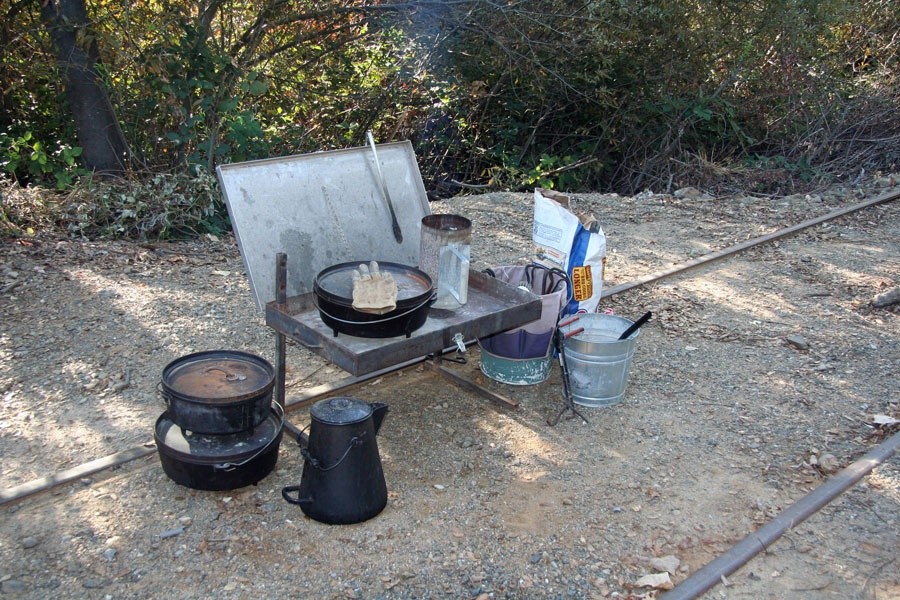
left=557, top=314, right=640, bottom=409
left=419, top=213, right=471, bottom=308
left=476, top=266, right=567, bottom=385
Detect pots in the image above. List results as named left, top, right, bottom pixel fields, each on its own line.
left=154, top=350, right=284, bottom=490
left=313, top=260, right=436, bottom=339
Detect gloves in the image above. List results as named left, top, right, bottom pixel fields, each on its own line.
left=352, top=260, right=398, bottom=315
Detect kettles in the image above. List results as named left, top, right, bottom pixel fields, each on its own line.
left=297, top=397, right=390, bottom=524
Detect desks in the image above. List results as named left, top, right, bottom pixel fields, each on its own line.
left=216, top=139, right=543, bottom=449
